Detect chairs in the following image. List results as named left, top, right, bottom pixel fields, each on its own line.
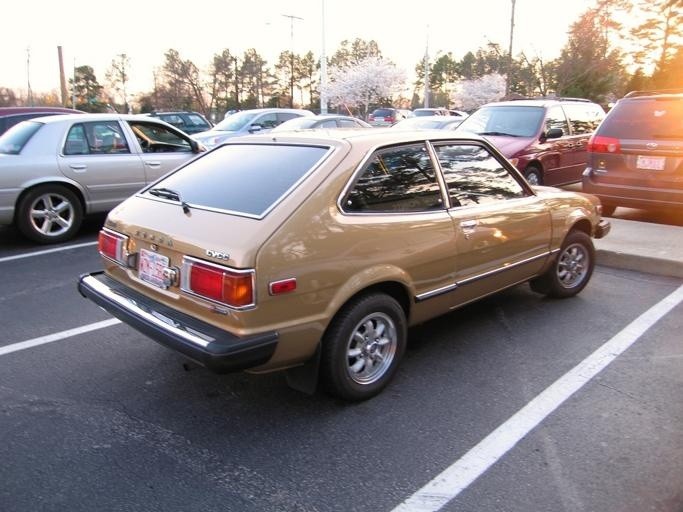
left=101, top=135, right=116, bottom=154
